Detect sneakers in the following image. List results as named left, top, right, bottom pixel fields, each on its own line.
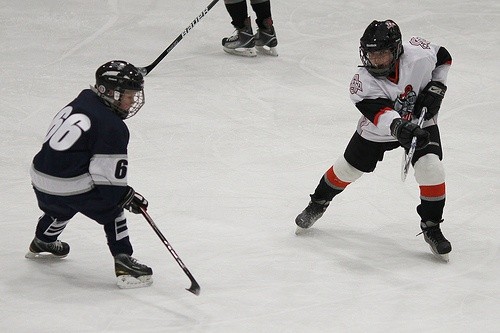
left=222, top=15, right=257, bottom=57
left=416, top=204, right=452, bottom=265
left=24, top=230, right=70, bottom=259
left=254, top=18, right=279, bottom=57
left=114, top=253, right=153, bottom=288
left=294, top=194, right=330, bottom=235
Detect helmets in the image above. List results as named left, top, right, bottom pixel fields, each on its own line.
left=358, top=19, right=404, bottom=80
left=95, top=61, right=146, bottom=120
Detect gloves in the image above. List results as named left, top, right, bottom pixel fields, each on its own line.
left=412, top=81, right=447, bottom=121
left=390, top=118, right=427, bottom=153
left=118, top=185, right=149, bottom=214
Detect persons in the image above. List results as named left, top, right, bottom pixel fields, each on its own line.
left=295, top=19, right=452, bottom=254
left=222, top=0, right=277, bottom=49
left=29, top=59, right=153, bottom=278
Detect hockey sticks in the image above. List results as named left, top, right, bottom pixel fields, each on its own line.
left=400, top=107, right=428, bottom=182
left=135, top=0, right=219, bottom=77
left=139, top=206, right=200, bottom=295
